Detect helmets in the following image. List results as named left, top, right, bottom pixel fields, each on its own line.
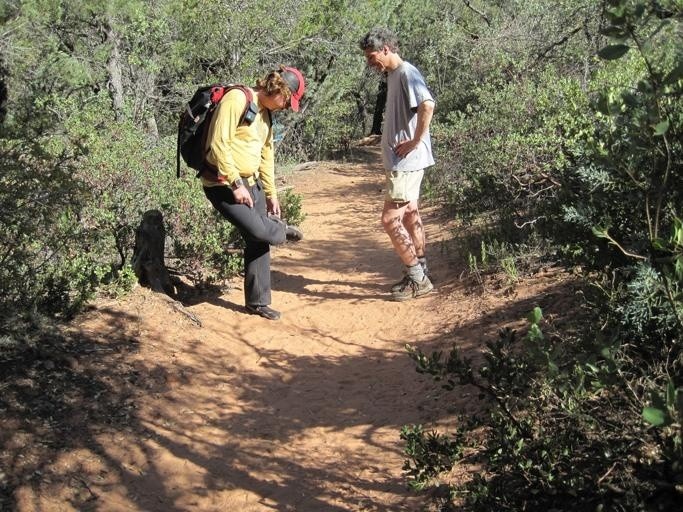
left=278, top=63, right=305, bottom=113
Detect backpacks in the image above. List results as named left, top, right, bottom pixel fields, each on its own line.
left=178, top=82, right=259, bottom=179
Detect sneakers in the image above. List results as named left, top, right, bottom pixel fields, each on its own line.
left=390, top=269, right=435, bottom=303
left=244, top=303, right=281, bottom=321
left=269, top=216, right=303, bottom=242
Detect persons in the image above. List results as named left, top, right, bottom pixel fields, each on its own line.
left=357, top=26, right=437, bottom=302
left=198, top=64, right=303, bottom=320
